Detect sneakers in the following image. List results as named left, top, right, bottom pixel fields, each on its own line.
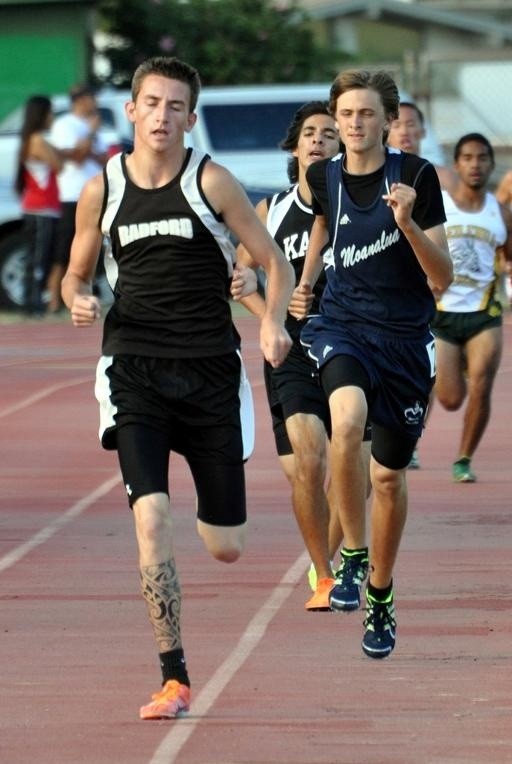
left=138, top=680, right=191, bottom=718
left=306, top=562, right=336, bottom=591
left=452, top=457, right=476, bottom=482
left=304, top=576, right=336, bottom=611
left=363, top=585, right=397, bottom=660
left=408, top=450, right=420, bottom=470
left=328, top=547, right=368, bottom=613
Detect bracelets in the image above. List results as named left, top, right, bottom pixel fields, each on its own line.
left=86, top=130, right=95, bottom=140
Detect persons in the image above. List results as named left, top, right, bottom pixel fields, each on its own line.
left=286, top=67, right=452, bottom=661
left=12, top=93, right=61, bottom=317
left=47, top=80, right=122, bottom=316
left=384, top=99, right=438, bottom=469
left=56, top=52, right=296, bottom=724
left=230, top=97, right=372, bottom=611
left=423, top=129, right=511, bottom=485
